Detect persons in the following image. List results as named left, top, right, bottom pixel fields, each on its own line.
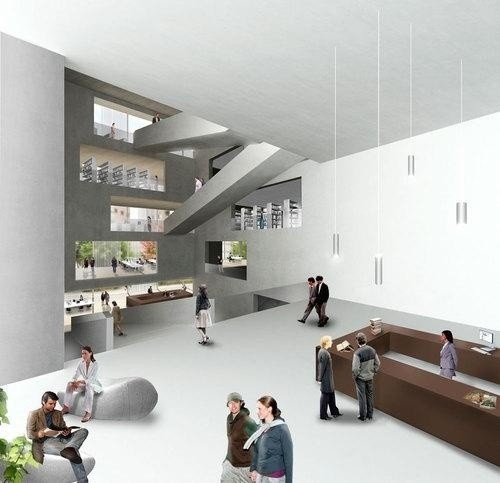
left=352, top=330, right=381, bottom=423
left=227, top=251, right=234, bottom=262
left=25, top=390, right=89, bottom=482
left=194, top=283, right=212, bottom=346
left=194, top=176, right=202, bottom=193
left=58, top=345, right=104, bottom=423
left=219, top=391, right=259, bottom=482
left=151, top=111, right=160, bottom=124
left=248, top=396, right=292, bottom=482
left=110, top=121, right=117, bottom=139
left=438, top=329, right=458, bottom=380
left=311, top=275, right=329, bottom=327
left=153, top=174, right=159, bottom=190
left=73, top=253, right=188, bottom=339
left=295, top=276, right=322, bottom=324
left=147, top=215, right=153, bottom=232
left=200, top=177, right=205, bottom=185
left=216, top=254, right=225, bottom=274
left=316, top=334, right=343, bottom=420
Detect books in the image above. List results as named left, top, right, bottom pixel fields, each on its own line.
left=369, top=316, right=383, bottom=336
left=336, top=338, right=354, bottom=356
left=42, top=425, right=81, bottom=441
left=471, top=344, right=496, bottom=358
left=466, top=389, right=498, bottom=410
